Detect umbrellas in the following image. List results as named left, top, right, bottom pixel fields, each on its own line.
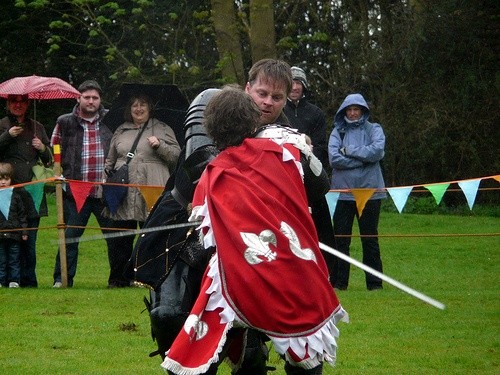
left=101, top=83, right=190, bottom=149
left=0, top=74, right=84, bottom=136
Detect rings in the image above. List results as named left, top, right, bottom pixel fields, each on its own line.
left=153, top=141, right=154, bottom=143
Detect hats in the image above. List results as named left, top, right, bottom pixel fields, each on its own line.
left=289, top=65, right=308, bottom=88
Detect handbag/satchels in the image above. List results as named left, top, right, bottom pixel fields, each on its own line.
left=103, top=165, right=130, bottom=214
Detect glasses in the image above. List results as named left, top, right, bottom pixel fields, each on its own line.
left=10, top=98, right=29, bottom=105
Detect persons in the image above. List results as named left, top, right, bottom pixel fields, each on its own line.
left=48, top=80, right=116, bottom=287
left=188, top=89, right=340, bottom=375
left=282, top=66, right=338, bottom=288
left=104, top=94, right=182, bottom=290
left=0, top=80, right=54, bottom=288
left=149, top=58, right=329, bottom=375
left=0, top=163, right=28, bottom=287
left=329, top=93, right=386, bottom=291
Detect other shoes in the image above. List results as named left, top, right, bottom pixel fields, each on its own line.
left=9, top=282, right=19, bottom=288
left=52, top=282, right=62, bottom=287
